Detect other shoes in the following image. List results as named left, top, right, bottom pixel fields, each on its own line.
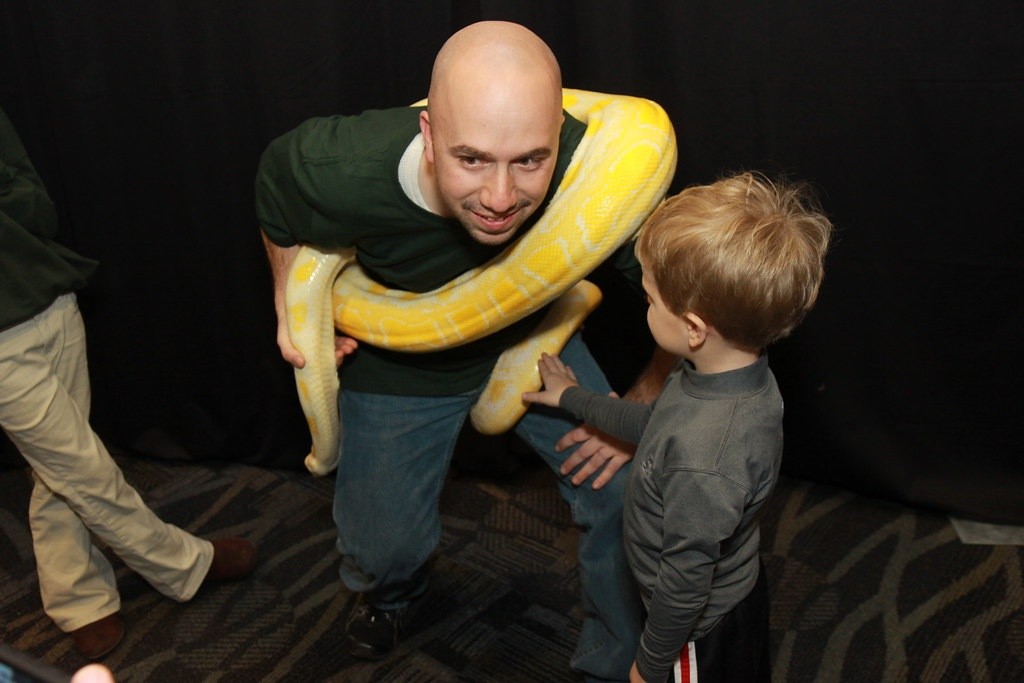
left=342, top=576, right=438, bottom=659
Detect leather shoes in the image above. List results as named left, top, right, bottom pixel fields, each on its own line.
left=69, top=613, right=125, bottom=660
left=204, top=536, right=256, bottom=581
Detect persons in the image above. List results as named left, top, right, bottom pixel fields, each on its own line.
left=250, top=18, right=688, bottom=683
left=519, top=172, right=834, bottom=683
left=0, top=105, right=258, bottom=658
